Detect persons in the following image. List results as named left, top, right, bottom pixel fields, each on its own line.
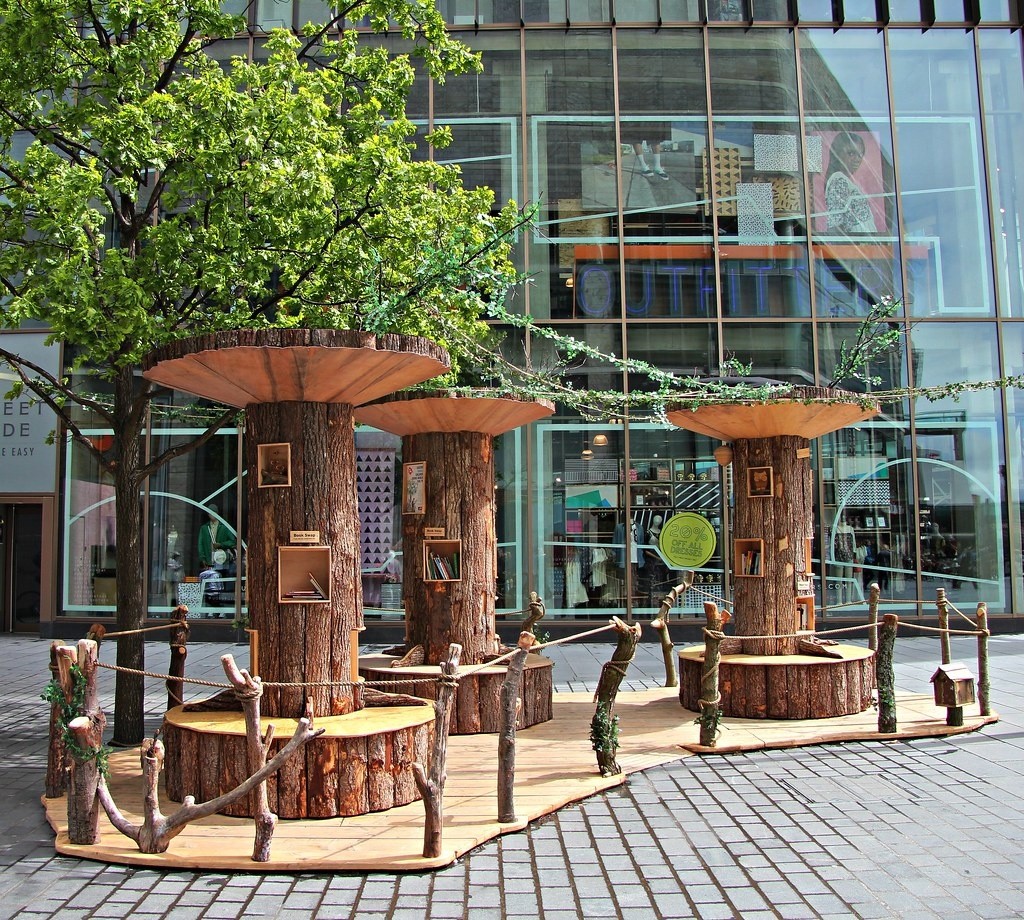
left=161, top=525, right=186, bottom=609
left=198, top=504, right=236, bottom=578
left=923, top=523, right=945, bottom=560
left=642, top=515, right=665, bottom=566
left=613, top=519, right=646, bottom=576
left=860, top=540, right=892, bottom=592
left=829, top=515, right=857, bottom=606
left=952, top=543, right=981, bottom=590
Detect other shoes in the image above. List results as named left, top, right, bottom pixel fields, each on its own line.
left=653, top=169, right=669, bottom=181
left=641, top=169, right=653, bottom=177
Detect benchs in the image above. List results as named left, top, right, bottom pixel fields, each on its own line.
left=357, top=646, right=553, bottom=733
left=678, top=643, right=878, bottom=719
left=157, top=689, right=445, bottom=818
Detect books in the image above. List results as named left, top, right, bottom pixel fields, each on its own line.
left=741, top=550, right=761, bottom=575
left=424, top=545, right=460, bottom=580
left=281, top=571, right=329, bottom=601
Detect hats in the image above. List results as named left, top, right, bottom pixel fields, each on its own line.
left=208, top=504, right=218, bottom=512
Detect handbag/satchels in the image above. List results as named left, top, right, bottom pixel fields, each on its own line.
left=214, top=549, right=238, bottom=565
